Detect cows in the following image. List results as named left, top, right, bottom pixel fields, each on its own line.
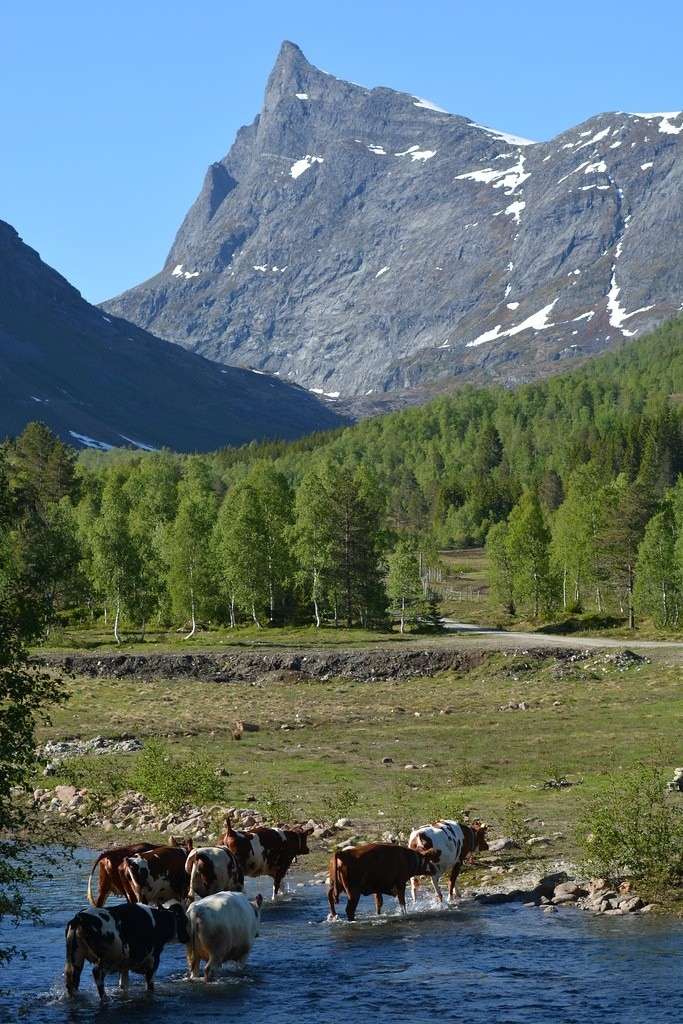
left=328, top=819, right=490, bottom=921
left=64, top=827, right=314, bottom=999
